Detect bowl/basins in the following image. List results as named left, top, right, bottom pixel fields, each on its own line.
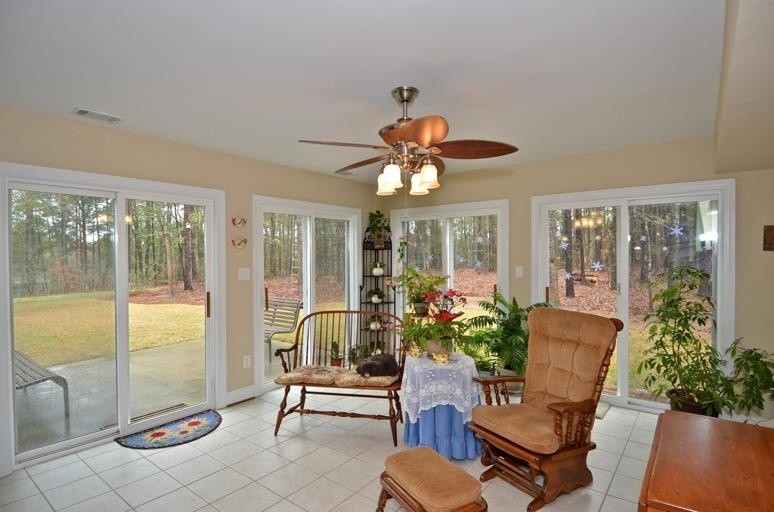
left=431, top=352, right=449, bottom=365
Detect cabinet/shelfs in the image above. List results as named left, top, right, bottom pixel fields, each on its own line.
left=355, top=219, right=400, bottom=363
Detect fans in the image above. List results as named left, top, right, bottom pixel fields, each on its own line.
left=295, top=83, right=523, bottom=179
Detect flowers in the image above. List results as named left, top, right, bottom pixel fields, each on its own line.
left=425, top=288, right=467, bottom=326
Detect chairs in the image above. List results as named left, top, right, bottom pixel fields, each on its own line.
left=464, top=302, right=626, bottom=512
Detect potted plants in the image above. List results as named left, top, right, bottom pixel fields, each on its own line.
left=467, top=288, right=556, bottom=396
left=367, top=261, right=386, bottom=277
left=389, top=313, right=470, bottom=359
left=329, top=342, right=343, bottom=367
left=633, top=253, right=774, bottom=420
left=382, top=264, right=451, bottom=315
left=369, top=341, right=382, bottom=356
left=365, top=287, right=385, bottom=305
left=365, top=315, right=386, bottom=332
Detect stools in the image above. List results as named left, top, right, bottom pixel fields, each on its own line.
left=376, top=445, right=492, bottom=512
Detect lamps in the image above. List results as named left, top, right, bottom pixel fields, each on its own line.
left=373, top=147, right=443, bottom=199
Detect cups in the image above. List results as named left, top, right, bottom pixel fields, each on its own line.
left=410, top=346, right=422, bottom=358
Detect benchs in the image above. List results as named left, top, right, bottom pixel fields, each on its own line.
left=11, top=347, right=71, bottom=420
left=264, top=294, right=300, bottom=364
left=274, top=305, right=412, bottom=449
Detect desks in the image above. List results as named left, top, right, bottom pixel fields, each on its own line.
left=630, top=406, right=773, bottom=511
left=398, top=348, right=486, bottom=461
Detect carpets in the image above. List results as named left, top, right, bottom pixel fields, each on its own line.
left=115, top=405, right=221, bottom=452
left=593, top=399, right=611, bottom=419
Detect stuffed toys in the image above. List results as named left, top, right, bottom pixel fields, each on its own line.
left=355, top=351, right=399, bottom=377
